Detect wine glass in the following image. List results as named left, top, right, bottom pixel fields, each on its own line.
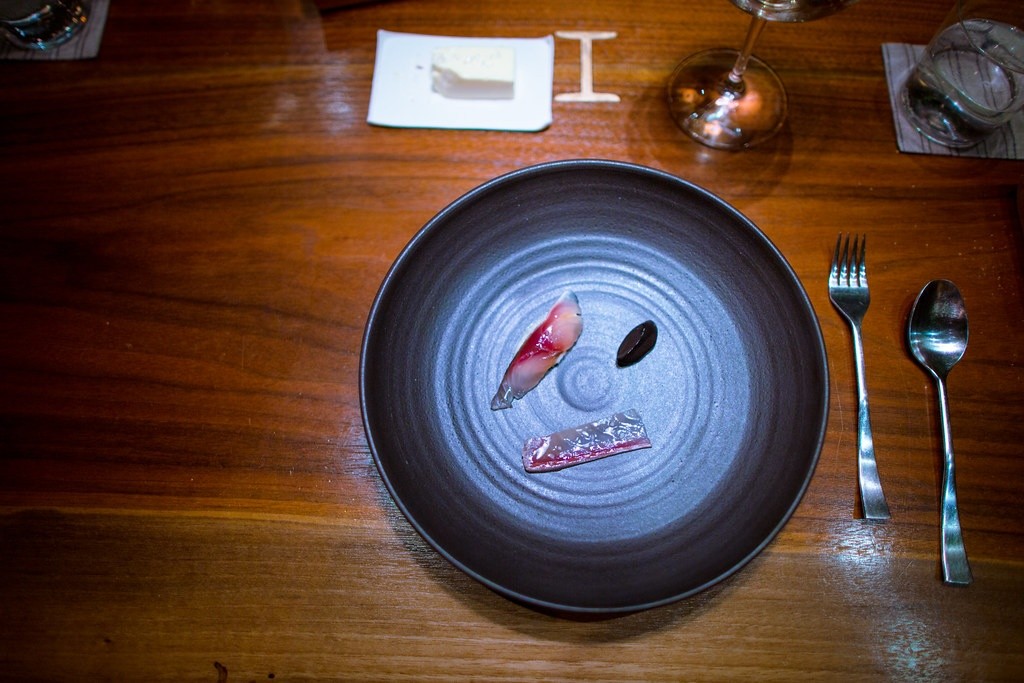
left=666, top=1, right=852, bottom=150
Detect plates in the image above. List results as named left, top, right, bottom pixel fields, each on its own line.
left=358, top=158, right=829, bottom=614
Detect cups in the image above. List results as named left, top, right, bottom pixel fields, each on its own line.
left=0, top=0, right=91, bottom=50
left=900, top=1, right=1024, bottom=147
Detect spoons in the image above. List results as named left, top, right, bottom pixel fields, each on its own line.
left=907, top=279, right=973, bottom=584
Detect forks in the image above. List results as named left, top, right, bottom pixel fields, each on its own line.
left=828, top=232, right=891, bottom=519
